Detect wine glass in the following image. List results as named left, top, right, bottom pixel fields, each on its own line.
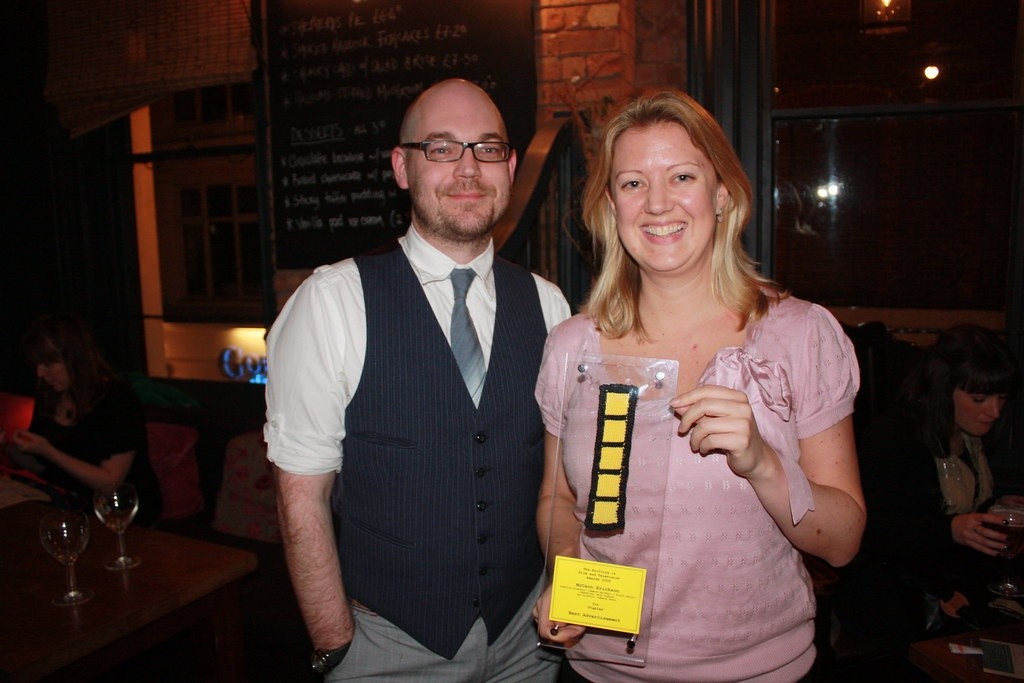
left=39, top=510, right=93, bottom=608
left=93, top=483, right=140, bottom=570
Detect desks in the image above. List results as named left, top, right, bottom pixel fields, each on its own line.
left=0, top=464, right=258, bottom=683
left=908, top=631, right=1024, bottom=683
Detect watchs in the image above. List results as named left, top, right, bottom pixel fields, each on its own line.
left=311, top=642, right=350, bottom=676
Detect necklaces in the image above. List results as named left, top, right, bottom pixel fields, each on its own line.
left=62, top=407, right=73, bottom=419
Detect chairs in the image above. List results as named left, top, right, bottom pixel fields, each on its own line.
left=809, top=338, right=1024, bottom=683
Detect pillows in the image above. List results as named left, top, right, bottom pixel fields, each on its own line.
left=141, top=423, right=201, bottom=518
left=211, top=434, right=284, bottom=543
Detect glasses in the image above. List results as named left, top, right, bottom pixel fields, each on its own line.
left=400, top=141, right=511, bottom=162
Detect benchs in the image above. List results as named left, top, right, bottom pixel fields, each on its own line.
left=104, top=375, right=341, bottom=640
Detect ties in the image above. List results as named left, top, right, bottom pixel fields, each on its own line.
left=449, top=268, right=486, bottom=410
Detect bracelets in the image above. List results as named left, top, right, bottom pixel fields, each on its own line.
left=50, top=448, right=69, bottom=472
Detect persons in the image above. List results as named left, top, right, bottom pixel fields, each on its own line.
left=263, top=74, right=575, bottom=683
left=0, top=314, right=161, bottom=532
left=863, top=324, right=1024, bottom=642
left=533, top=86, right=868, bottom=683
